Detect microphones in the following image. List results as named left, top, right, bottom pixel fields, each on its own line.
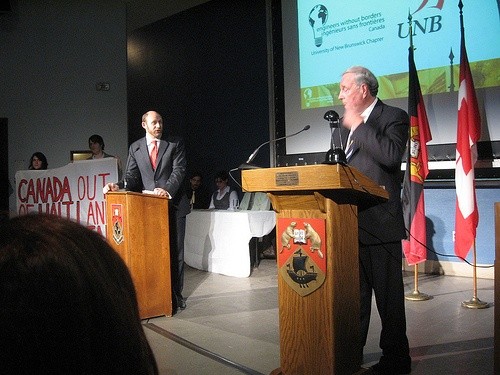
left=119, top=147, right=140, bottom=192
left=238, top=124, right=310, bottom=170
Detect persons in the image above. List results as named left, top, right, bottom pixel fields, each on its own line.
left=187, top=172, right=203, bottom=209
left=240, top=191, right=271, bottom=267
left=86, top=134, right=124, bottom=190
left=0, top=212, right=159, bottom=375
left=27, top=151, right=48, bottom=170
left=208, top=172, right=239, bottom=210
left=330, top=66, right=413, bottom=375
left=103, top=111, right=191, bottom=310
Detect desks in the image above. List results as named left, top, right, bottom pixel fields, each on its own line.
left=184, top=210, right=276, bottom=278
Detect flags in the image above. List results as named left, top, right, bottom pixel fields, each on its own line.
left=403, top=50, right=432, bottom=267
left=454, top=32, right=481, bottom=259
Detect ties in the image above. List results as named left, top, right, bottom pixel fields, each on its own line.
left=190, top=191, right=195, bottom=203
left=345, top=134, right=355, bottom=161
left=150, top=140, right=158, bottom=168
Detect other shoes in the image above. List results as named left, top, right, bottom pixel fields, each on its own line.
left=177, top=295, right=186, bottom=310
left=361, top=362, right=412, bottom=375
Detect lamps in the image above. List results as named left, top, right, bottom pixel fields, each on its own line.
left=324, top=111, right=347, bottom=166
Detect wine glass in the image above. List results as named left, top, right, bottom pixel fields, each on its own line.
left=233, top=199, right=239, bottom=211
